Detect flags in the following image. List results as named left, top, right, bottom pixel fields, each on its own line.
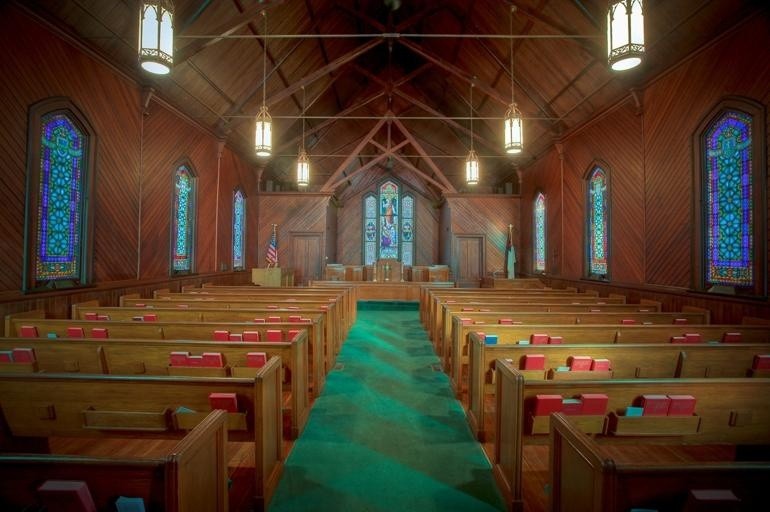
left=507, top=232, right=517, bottom=280
left=264, top=229, right=278, bottom=269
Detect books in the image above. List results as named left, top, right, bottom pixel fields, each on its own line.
left=752, top=353, right=770, bottom=369
left=131, top=317, right=144, bottom=321
left=579, top=393, right=609, bottom=414
left=590, top=357, right=610, bottom=371
left=477, top=332, right=486, bottom=340
left=84, top=312, right=98, bottom=321
left=40, top=479, right=96, bottom=511
left=640, top=394, right=669, bottom=416
left=671, top=318, right=689, bottom=325
left=667, top=394, right=697, bottom=417
left=48, top=333, right=57, bottom=338
left=474, top=319, right=487, bottom=324
left=114, top=495, right=146, bottom=512
left=548, top=336, right=563, bottom=344
left=670, top=336, right=689, bottom=344
left=625, top=407, right=645, bottom=417
left=446, top=300, right=457, bottom=303
left=555, top=365, right=571, bottom=372
left=169, top=291, right=331, bottom=413
left=684, top=332, right=701, bottom=342
left=530, top=333, right=549, bottom=344
left=534, top=394, right=563, bottom=414
left=67, top=326, right=86, bottom=338
left=0, top=349, right=14, bottom=363
left=478, top=308, right=492, bottom=312
left=144, top=313, right=158, bottom=322
left=460, top=317, right=473, bottom=324
left=568, top=354, right=593, bottom=370
left=484, top=334, right=499, bottom=344
left=638, top=309, right=649, bottom=311
left=20, top=325, right=39, bottom=338
left=523, top=353, right=545, bottom=369
left=462, top=308, right=476, bottom=311
left=135, top=302, right=147, bottom=307
left=622, top=319, right=636, bottom=325
left=643, top=321, right=655, bottom=325
left=590, top=307, right=602, bottom=312
left=498, top=319, right=515, bottom=325
left=98, top=315, right=111, bottom=321
left=596, top=300, right=609, bottom=304
left=511, top=321, right=524, bottom=325
left=722, top=330, right=743, bottom=343
left=92, top=327, right=108, bottom=338
left=12, top=347, right=36, bottom=364
left=571, top=301, right=583, bottom=304
left=560, top=397, right=583, bottom=415
left=517, top=340, right=531, bottom=345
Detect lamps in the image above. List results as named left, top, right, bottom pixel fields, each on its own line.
left=465, top=81, right=480, bottom=185
left=295, top=84, right=310, bottom=186
left=254, top=8, right=274, bottom=158
left=137, top=0, right=173, bottom=76
left=503, top=4, right=524, bottom=155
